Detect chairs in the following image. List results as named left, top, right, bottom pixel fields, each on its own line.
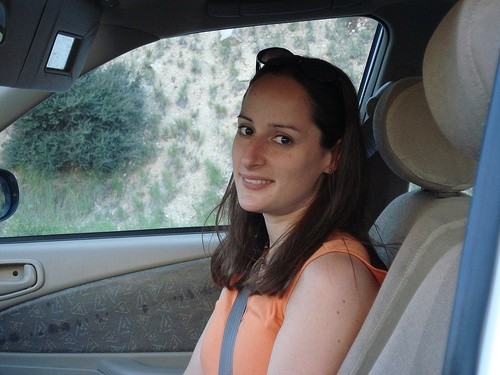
left=337, top=77, right=480, bottom=375
left=353, top=0, right=500, bottom=375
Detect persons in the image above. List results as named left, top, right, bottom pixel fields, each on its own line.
left=180, top=48, right=392, bottom=374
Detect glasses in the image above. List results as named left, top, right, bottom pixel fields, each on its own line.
left=257, top=47, right=336, bottom=83
